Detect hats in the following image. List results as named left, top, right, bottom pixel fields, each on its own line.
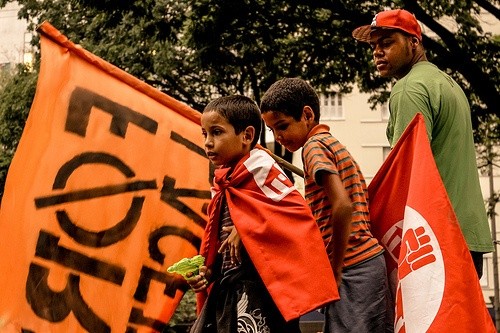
left=352, top=8, right=423, bottom=44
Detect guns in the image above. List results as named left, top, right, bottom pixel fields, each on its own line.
left=167, top=254, right=208, bottom=289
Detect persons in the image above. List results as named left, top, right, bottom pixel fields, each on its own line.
left=354, top=9, right=496, bottom=285
left=257, top=78, right=393, bottom=332
left=175, top=93, right=341, bottom=333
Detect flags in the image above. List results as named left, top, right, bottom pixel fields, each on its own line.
left=0, top=22, right=272, bottom=333
left=342, top=112, right=498, bottom=332
